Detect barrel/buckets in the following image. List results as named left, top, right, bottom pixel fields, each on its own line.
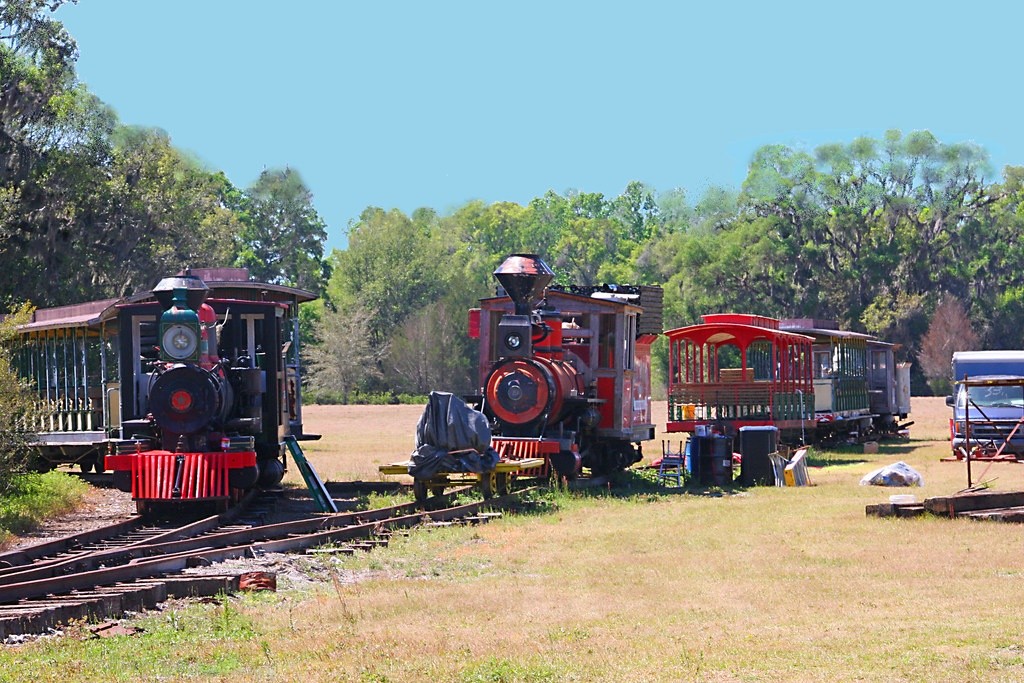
left=889, top=494, right=916, bottom=510
left=687, top=436, right=733, bottom=485
left=681, top=404, right=695, bottom=420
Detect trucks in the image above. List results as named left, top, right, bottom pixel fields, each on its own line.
left=944, top=350, right=1024, bottom=461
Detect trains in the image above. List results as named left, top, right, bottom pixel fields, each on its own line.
left=0, top=265, right=322, bottom=514
left=662, top=313, right=916, bottom=448
left=379, top=256, right=656, bottom=505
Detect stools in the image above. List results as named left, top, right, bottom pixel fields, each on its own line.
left=658, top=440, right=688, bottom=493
